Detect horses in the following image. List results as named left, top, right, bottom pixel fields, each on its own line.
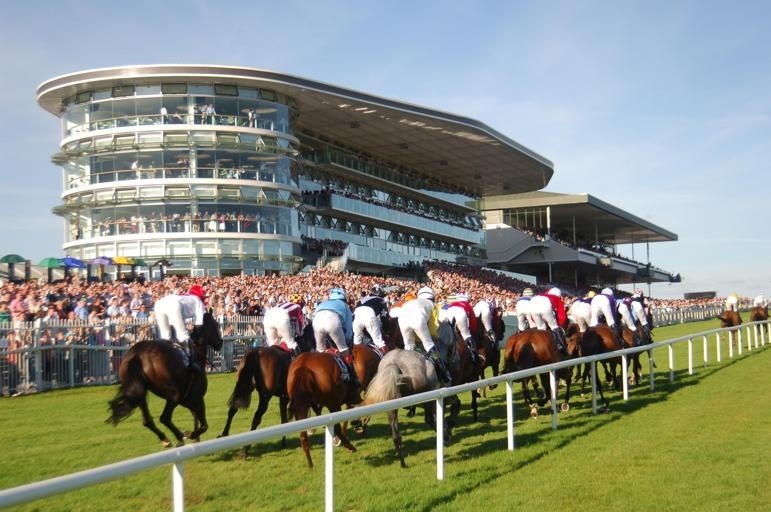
left=749, top=304, right=768, bottom=336
left=103, top=306, right=224, bottom=448
left=216, top=306, right=657, bottom=471
left=716, top=305, right=743, bottom=349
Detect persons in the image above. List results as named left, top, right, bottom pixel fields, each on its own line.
left=0, top=102, right=771, bottom=390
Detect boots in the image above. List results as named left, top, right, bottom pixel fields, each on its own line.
left=611, top=324, right=626, bottom=345
left=182, top=338, right=202, bottom=373
left=464, top=337, right=478, bottom=363
left=341, top=349, right=361, bottom=388
left=553, top=328, right=567, bottom=352
left=428, top=346, right=451, bottom=383
left=489, top=329, right=498, bottom=346
left=633, top=328, right=645, bottom=345
left=643, top=323, right=654, bottom=342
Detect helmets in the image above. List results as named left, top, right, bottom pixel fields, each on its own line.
left=328, top=288, right=347, bottom=299
left=523, top=288, right=534, bottom=296
left=290, top=293, right=306, bottom=308
left=601, top=287, right=612, bottom=295
left=190, top=286, right=207, bottom=299
left=417, top=285, right=434, bottom=300
left=586, top=291, right=596, bottom=298
left=370, top=284, right=387, bottom=296
left=548, top=287, right=561, bottom=297
